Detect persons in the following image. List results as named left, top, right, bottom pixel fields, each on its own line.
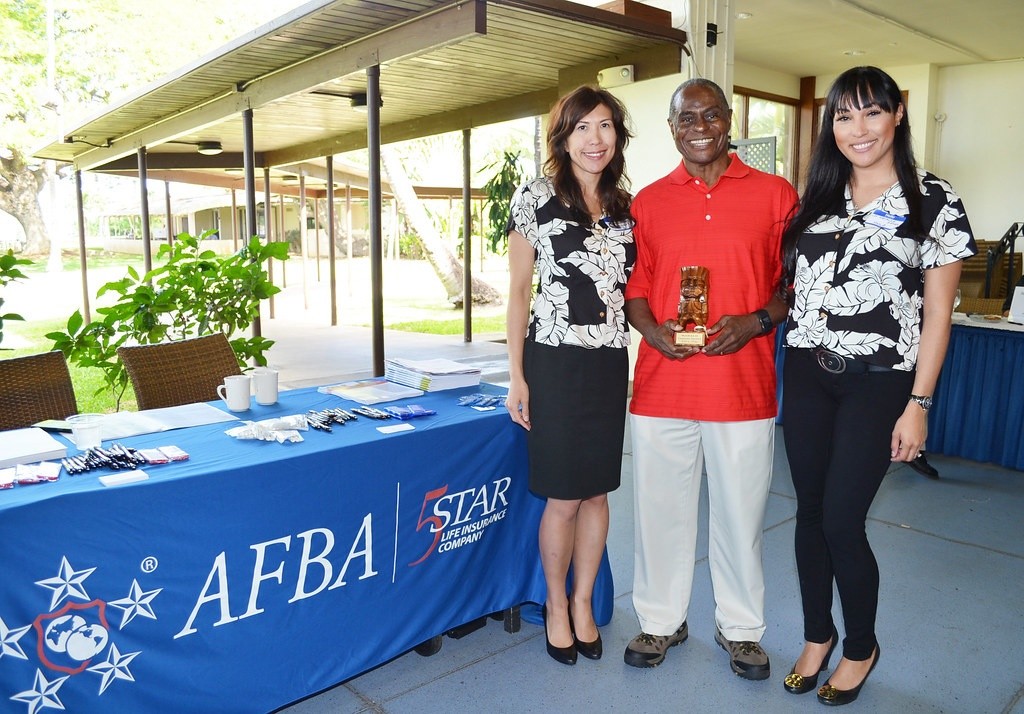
left=785, top=66, right=979, bottom=706
left=900, top=439, right=938, bottom=480
left=1002, top=274, right=1024, bottom=316
left=622, top=80, right=800, bottom=681
left=507, top=85, right=639, bottom=666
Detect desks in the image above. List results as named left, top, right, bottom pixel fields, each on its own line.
left=0, top=376, right=616, bottom=714
left=770, top=311, right=1024, bottom=472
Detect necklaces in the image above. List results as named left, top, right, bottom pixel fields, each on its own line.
left=853, top=193, right=880, bottom=211
left=591, top=216, right=596, bottom=218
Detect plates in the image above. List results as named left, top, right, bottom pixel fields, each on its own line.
left=969, top=315, right=1005, bottom=322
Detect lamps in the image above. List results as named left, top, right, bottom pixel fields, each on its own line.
left=311, top=91, right=385, bottom=111
left=167, top=138, right=223, bottom=156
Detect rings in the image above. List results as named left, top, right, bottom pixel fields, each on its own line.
left=918, top=453, right=922, bottom=457
left=720, top=351, right=723, bottom=355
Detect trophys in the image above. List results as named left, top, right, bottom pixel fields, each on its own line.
left=674, top=265, right=711, bottom=346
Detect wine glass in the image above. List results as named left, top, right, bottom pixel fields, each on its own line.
left=952, top=289, right=962, bottom=315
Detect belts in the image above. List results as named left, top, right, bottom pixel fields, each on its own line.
left=796, top=346, right=897, bottom=375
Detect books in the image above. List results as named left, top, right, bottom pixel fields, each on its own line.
left=317, top=357, right=482, bottom=405
left=0, top=428, right=67, bottom=469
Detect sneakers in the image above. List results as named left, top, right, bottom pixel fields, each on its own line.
left=626, top=619, right=688, bottom=667
left=713, top=624, right=770, bottom=679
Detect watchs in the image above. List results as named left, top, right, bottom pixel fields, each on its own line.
left=909, top=395, right=932, bottom=411
left=751, top=309, right=773, bottom=335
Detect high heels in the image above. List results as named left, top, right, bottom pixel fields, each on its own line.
left=817, top=645, right=881, bottom=705
left=784, top=626, right=839, bottom=694
left=568, top=601, right=604, bottom=660
left=541, top=599, right=576, bottom=665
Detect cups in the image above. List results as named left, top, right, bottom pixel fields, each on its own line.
left=66, top=413, right=107, bottom=451
left=216, top=375, right=252, bottom=413
left=252, top=368, right=279, bottom=405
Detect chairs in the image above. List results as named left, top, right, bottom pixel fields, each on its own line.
left=0, top=348, right=78, bottom=433
left=116, top=334, right=245, bottom=413
left=955, top=298, right=1006, bottom=315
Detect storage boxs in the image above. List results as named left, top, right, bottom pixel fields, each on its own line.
left=1007, top=286, right=1024, bottom=325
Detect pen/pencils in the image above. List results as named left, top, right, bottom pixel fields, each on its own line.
left=307, top=405, right=391, bottom=432
left=63, top=441, right=147, bottom=474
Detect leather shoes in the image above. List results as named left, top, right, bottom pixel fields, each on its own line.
left=898, top=450, right=939, bottom=478
